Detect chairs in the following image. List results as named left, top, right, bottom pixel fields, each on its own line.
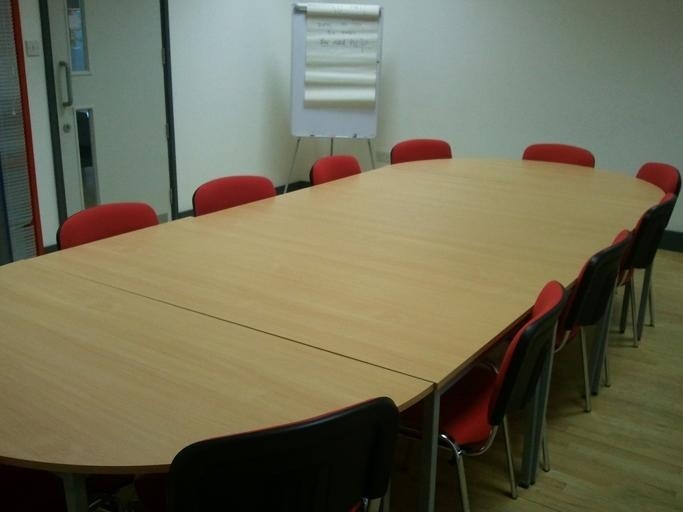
left=523, top=144, right=594, bottom=168
left=400, top=279, right=567, bottom=509
left=636, top=162, right=680, bottom=327
left=56, top=202, right=159, bottom=252
left=506, top=227, right=634, bottom=473
left=168, top=395, right=403, bottom=511
left=192, top=176, right=279, bottom=218
left=309, top=156, right=360, bottom=186
left=616, top=192, right=678, bottom=333
left=390, top=139, right=451, bottom=166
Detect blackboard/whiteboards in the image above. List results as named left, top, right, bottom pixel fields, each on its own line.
left=292, top=3, right=381, bottom=138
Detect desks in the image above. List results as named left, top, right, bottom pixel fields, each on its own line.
left=2, top=158, right=666, bottom=510
